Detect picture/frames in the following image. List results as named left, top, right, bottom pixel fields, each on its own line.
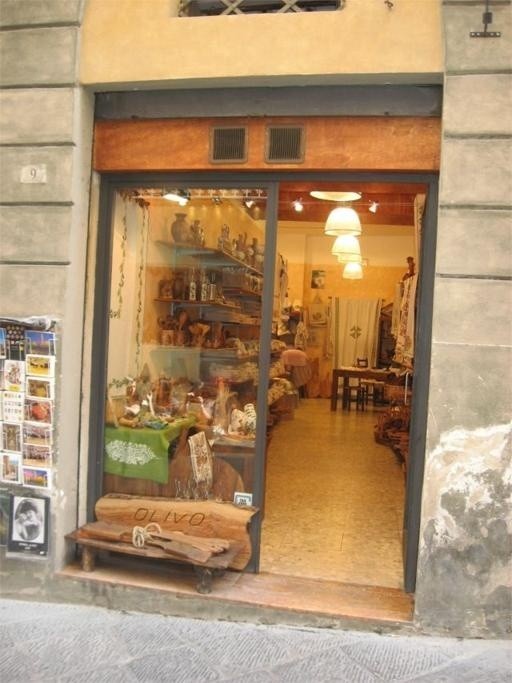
left=330, top=366, right=387, bottom=413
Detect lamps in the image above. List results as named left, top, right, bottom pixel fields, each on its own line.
left=357, top=356, right=385, bottom=407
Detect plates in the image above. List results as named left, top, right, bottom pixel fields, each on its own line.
left=152, top=238, right=289, bottom=361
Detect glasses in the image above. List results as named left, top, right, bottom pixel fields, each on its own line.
left=162, top=187, right=188, bottom=204
left=307, top=188, right=366, bottom=285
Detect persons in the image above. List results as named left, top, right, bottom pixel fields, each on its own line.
left=13, top=501, right=41, bottom=542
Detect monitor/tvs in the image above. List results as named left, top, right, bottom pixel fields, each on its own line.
left=0, top=322, right=55, bottom=491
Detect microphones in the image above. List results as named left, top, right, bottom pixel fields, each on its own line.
left=62, top=519, right=246, bottom=595
left=344, top=386, right=365, bottom=413
